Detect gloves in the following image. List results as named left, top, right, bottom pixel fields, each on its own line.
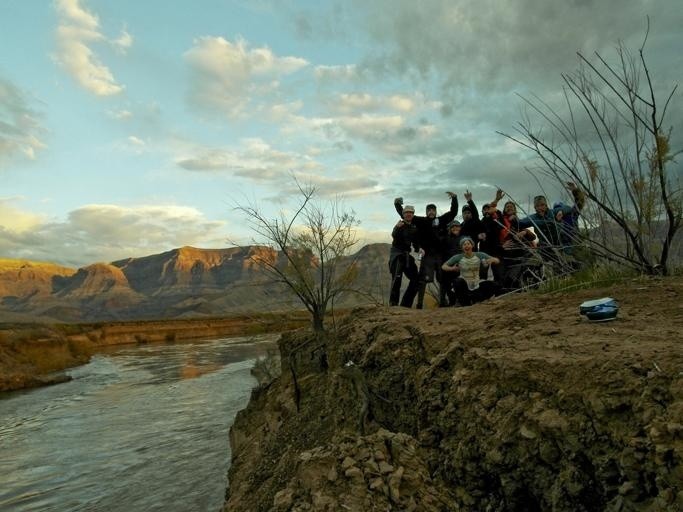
left=395, top=197, right=403, bottom=205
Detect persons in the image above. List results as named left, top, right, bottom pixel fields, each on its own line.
left=387, top=180, right=584, bottom=310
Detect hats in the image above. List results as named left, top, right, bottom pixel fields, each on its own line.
left=426, top=204, right=436, bottom=209
left=402, top=206, right=414, bottom=215
